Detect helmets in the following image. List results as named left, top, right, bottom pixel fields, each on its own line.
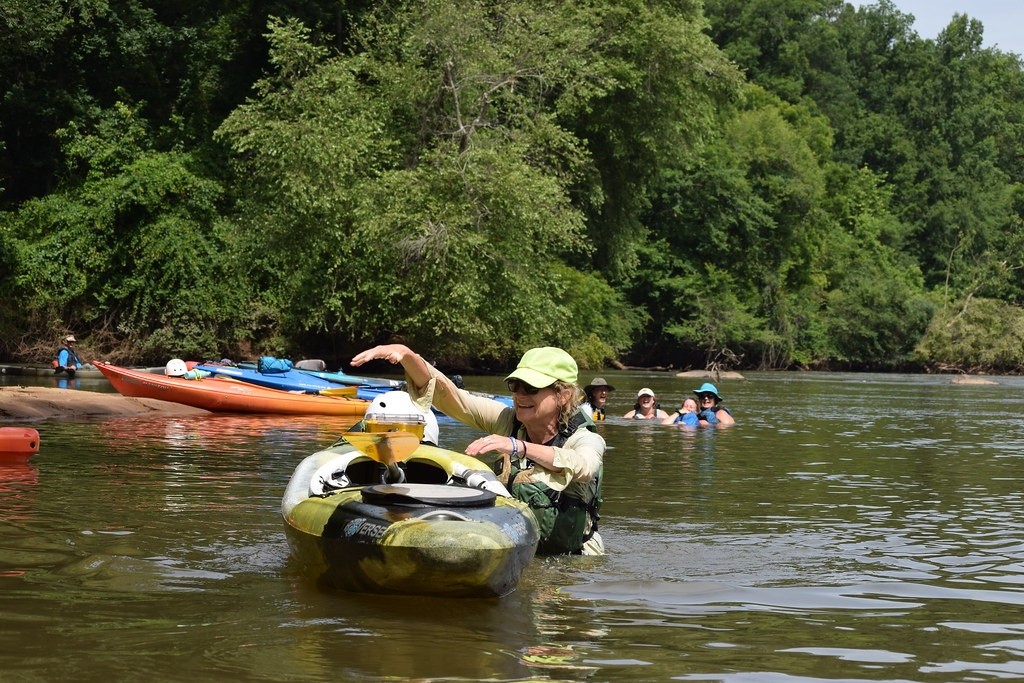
left=364, top=389, right=439, bottom=446
left=164, top=359, right=187, bottom=376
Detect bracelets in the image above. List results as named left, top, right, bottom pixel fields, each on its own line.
left=508, top=436, right=527, bottom=460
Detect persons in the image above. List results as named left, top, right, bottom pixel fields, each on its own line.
left=350, top=344, right=608, bottom=557
left=622, top=383, right=734, bottom=424
left=580, top=377, right=615, bottom=421
left=57, top=335, right=81, bottom=370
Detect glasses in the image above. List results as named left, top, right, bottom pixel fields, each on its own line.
left=698, top=394, right=717, bottom=399
left=507, top=380, right=557, bottom=396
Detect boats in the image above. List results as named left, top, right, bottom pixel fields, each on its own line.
left=191, top=357, right=515, bottom=418
left=90, top=357, right=374, bottom=417
left=279, top=390, right=541, bottom=601
left=235, top=359, right=406, bottom=390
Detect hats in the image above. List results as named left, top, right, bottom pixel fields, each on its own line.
left=692, top=383, right=722, bottom=402
left=503, top=346, right=578, bottom=389
left=638, top=388, right=655, bottom=399
left=583, top=377, right=616, bottom=396
left=66, top=336, right=76, bottom=342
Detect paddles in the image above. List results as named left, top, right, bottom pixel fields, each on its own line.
left=339, top=429, right=421, bottom=484
left=289, top=386, right=358, bottom=400
